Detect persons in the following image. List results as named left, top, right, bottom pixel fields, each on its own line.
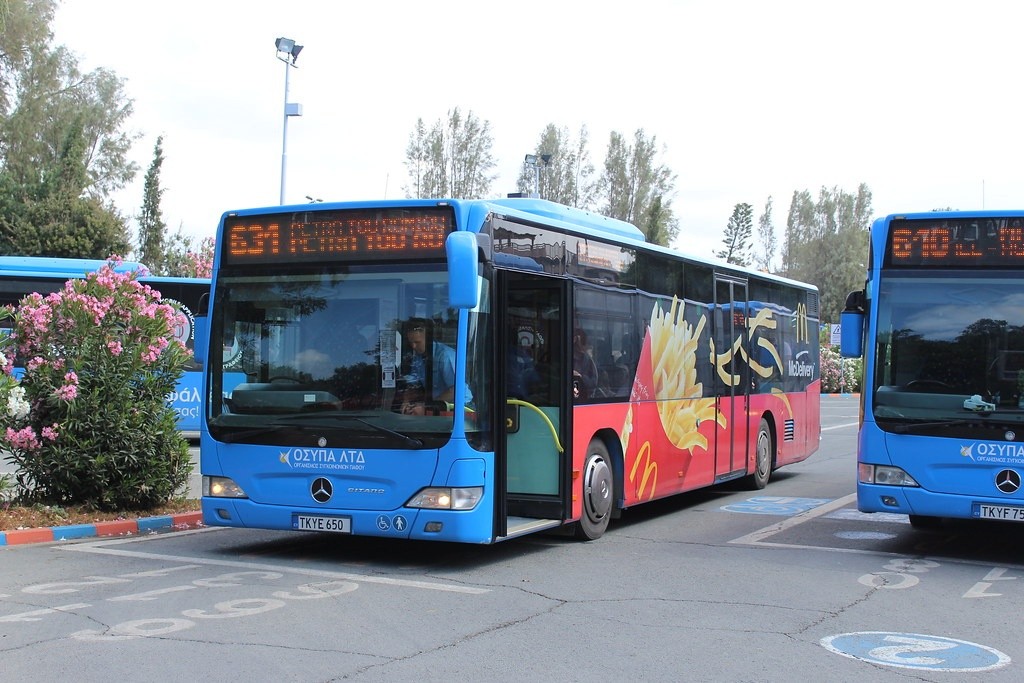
left=399, top=323, right=476, bottom=417
left=296, top=303, right=367, bottom=396
left=572, top=324, right=642, bottom=399
left=755, top=327, right=810, bottom=383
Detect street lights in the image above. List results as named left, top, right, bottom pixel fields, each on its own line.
left=272, top=33, right=303, bottom=202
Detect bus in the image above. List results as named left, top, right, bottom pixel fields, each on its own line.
left=189, top=194, right=822, bottom=546
left=839, top=208, right=1020, bottom=529
left=0, top=254, right=279, bottom=450
